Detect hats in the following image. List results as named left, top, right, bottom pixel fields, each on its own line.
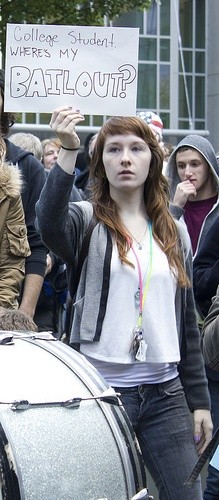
left=137, top=112, right=163, bottom=136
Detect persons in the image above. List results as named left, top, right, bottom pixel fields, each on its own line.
left=201, top=287, right=219, bottom=500
left=0, top=70, right=219, bottom=332
left=36, top=105, right=215, bottom=500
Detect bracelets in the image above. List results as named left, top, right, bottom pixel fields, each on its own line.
left=60, top=145, right=80, bottom=152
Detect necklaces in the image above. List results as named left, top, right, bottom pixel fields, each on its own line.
left=122, top=222, right=149, bottom=249
left=133, top=229, right=154, bottom=308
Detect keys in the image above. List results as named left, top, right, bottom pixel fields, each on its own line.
left=130, top=327, right=148, bottom=362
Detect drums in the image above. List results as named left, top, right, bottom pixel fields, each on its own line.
left=0, top=330, right=155, bottom=500
